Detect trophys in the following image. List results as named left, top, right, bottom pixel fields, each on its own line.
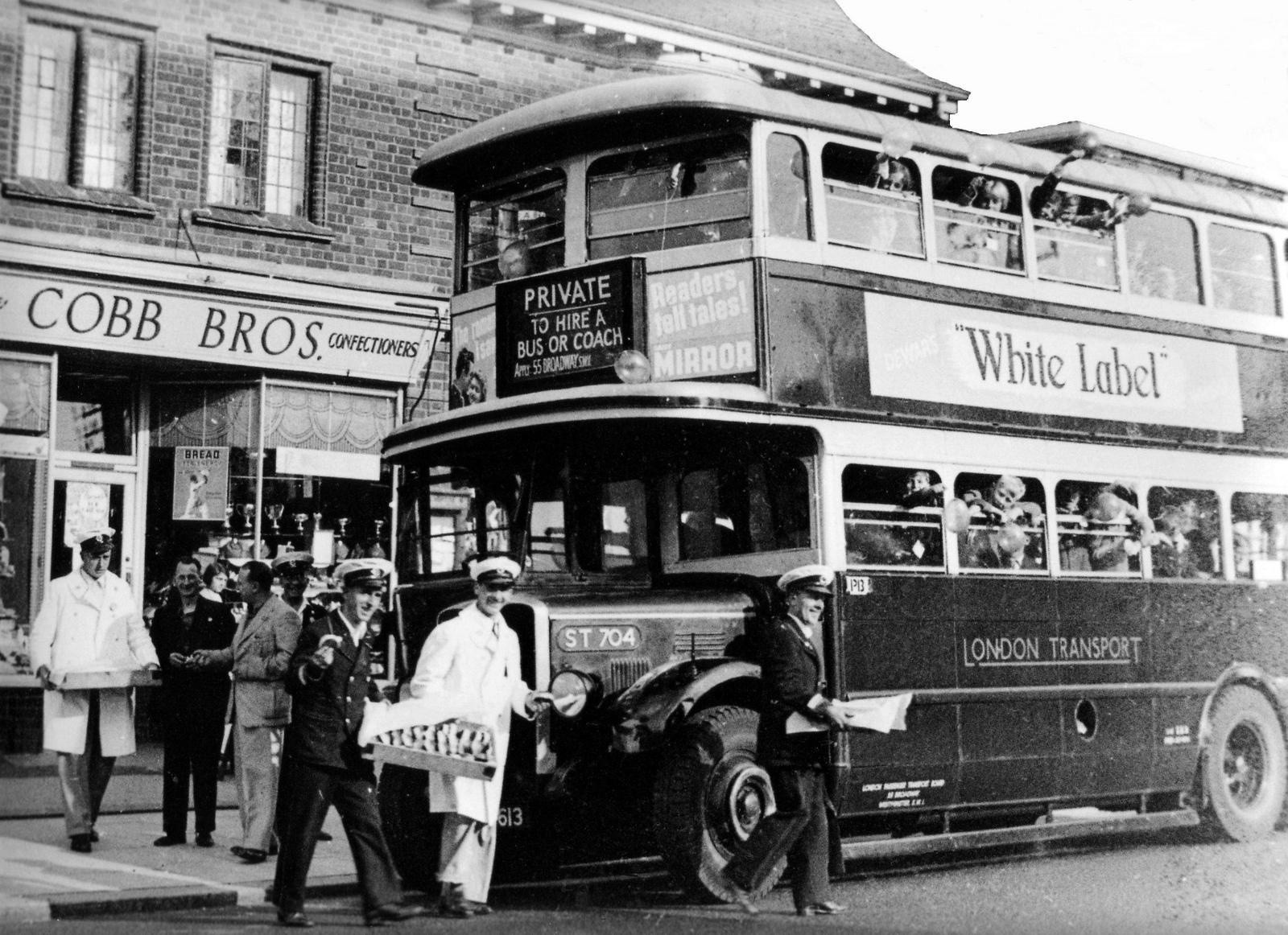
left=223, top=502, right=308, bottom=530
left=313, top=513, right=322, bottom=529
left=333, top=516, right=351, bottom=532
left=374, top=520, right=383, bottom=534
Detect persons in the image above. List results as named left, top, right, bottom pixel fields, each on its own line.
left=901, top=471, right=1288, bottom=577
left=199, top=562, right=230, bottom=603
left=29, top=526, right=161, bottom=854
left=191, top=561, right=302, bottom=861
left=716, top=564, right=848, bottom=918
left=851, top=148, right=1178, bottom=299
left=268, top=557, right=548, bottom=927
left=271, top=552, right=336, bottom=624
left=149, top=556, right=237, bottom=847
left=183, top=472, right=210, bottom=519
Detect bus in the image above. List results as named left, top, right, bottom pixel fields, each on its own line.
left=382, top=71, right=1288, bottom=908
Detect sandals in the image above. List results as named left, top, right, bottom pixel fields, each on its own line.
left=317, top=830, right=333, bottom=841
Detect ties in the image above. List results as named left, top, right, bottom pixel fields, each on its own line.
left=492, top=623, right=499, bottom=637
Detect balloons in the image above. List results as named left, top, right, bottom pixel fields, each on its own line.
left=882, top=125, right=913, bottom=158
left=1090, top=492, right=1123, bottom=522
left=966, top=139, right=995, bottom=170
left=944, top=498, right=972, bottom=532
left=1072, top=131, right=1151, bottom=216
left=998, top=521, right=1027, bottom=551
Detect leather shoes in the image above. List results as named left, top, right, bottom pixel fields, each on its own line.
left=720, top=868, right=759, bottom=915
left=364, top=902, right=423, bottom=926
left=278, top=906, right=314, bottom=926
left=800, top=900, right=847, bottom=916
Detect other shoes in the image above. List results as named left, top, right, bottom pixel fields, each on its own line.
left=71, top=835, right=92, bottom=852
left=230, top=846, right=267, bottom=861
left=153, top=832, right=187, bottom=846
left=196, top=831, right=214, bottom=846
left=437, top=898, right=473, bottom=915
left=468, top=902, right=492, bottom=913
left=269, top=838, right=279, bottom=853
left=90, top=829, right=99, bottom=840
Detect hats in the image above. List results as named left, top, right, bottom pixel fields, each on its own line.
left=471, top=557, right=523, bottom=585
left=333, top=558, right=389, bottom=588
left=74, top=527, right=116, bottom=554
left=777, top=564, right=837, bottom=598
left=271, top=551, right=314, bottom=575
